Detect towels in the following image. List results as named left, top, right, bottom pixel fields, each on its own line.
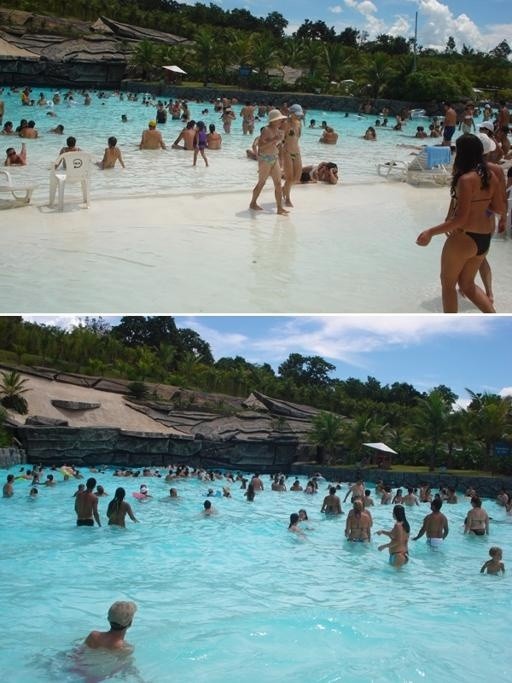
left=416, top=146, right=451, bottom=170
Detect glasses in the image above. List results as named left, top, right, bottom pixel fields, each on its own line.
left=8, top=151, right=16, bottom=157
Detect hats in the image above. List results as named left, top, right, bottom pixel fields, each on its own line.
left=268, top=109, right=288, bottom=123
left=289, top=103, right=304, bottom=118
left=108, top=600, right=138, bottom=628
left=475, top=133, right=497, bottom=155
left=463, top=100, right=492, bottom=109
left=149, top=119, right=157, bottom=127
left=476, top=120, right=496, bottom=135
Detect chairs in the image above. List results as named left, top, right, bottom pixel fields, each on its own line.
left=377, top=145, right=451, bottom=182
left=48, top=151, right=93, bottom=212
left=0, top=170, right=40, bottom=210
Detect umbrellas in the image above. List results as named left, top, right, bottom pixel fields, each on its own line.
left=161, top=65, right=187, bottom=75
left=361, top=442, right=398, bottom=465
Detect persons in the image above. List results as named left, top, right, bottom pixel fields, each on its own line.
left=50, top=601, right=144, bottom=683
